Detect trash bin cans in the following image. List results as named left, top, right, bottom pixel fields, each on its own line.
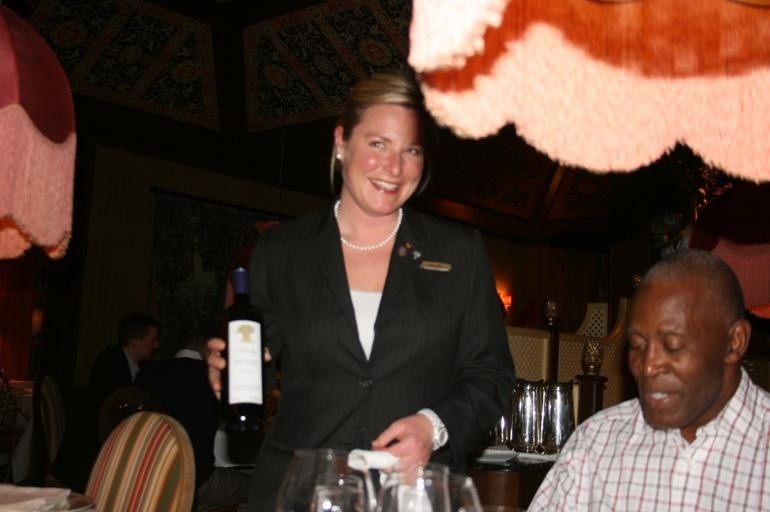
left=417, top=408, right=450, bottom=453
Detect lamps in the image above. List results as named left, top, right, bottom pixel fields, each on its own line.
left=85, top=410, right=196, bottom=511
left=29, top=370, right=65, bottom=487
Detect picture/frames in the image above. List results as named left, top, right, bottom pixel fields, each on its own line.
left=4, top=374, right=36, bottom=485
left=1, top=479, right=94, bottom=511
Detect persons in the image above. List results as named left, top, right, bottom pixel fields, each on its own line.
left=51, top=312, right=161, bottom=493
left=517, top=248, right=770, bottom=511
left=210, top=72, right=516, bottom=512
left=136, top=325, right=251, bottom=511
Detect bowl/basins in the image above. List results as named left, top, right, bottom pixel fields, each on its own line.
left=334, top=199, right=403, bottom=251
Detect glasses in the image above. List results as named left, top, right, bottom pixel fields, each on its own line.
left=404, top=0, right=767, bottom=186
left=0, top=0, right=77, bottom=264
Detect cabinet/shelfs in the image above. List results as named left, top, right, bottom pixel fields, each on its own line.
left=482, top=380, right=581, bottom=455
left=217, top=264, right=269, bottom=432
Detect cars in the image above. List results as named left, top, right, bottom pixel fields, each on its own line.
left=272, top=448, right=485, bottom=512
